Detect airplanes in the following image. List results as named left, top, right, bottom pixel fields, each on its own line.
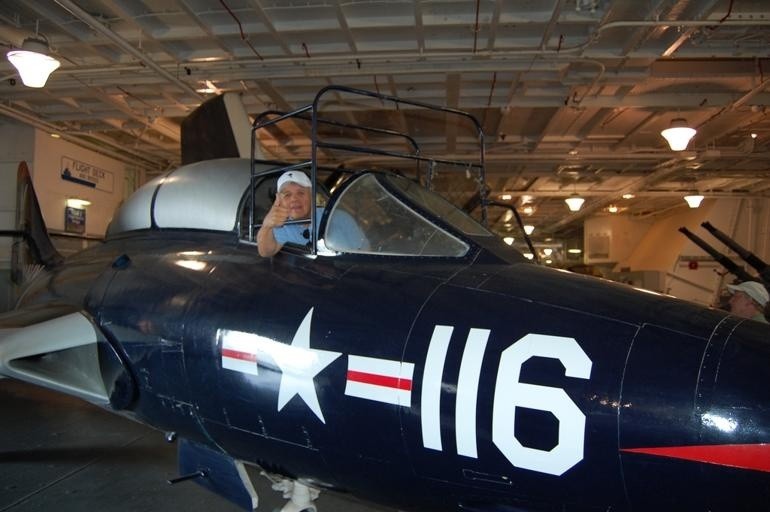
left=0, top=86, right=770, bottom=512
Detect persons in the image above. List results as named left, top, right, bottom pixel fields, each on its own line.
left=726, top=281, right=770, bottom=324
left=256, top=171, right=371, bottom=259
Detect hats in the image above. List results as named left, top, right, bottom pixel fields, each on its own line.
left=275, top=170, right=312, bottom=194
left=725, top=280, right=769, bottom=306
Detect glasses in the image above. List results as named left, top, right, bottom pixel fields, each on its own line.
left=303, top=227, right=313, bottom=249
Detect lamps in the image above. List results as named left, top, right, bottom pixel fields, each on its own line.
left=7, top=13, right=62, bottom=89
left=499, top=104, right=708, bottom=263
